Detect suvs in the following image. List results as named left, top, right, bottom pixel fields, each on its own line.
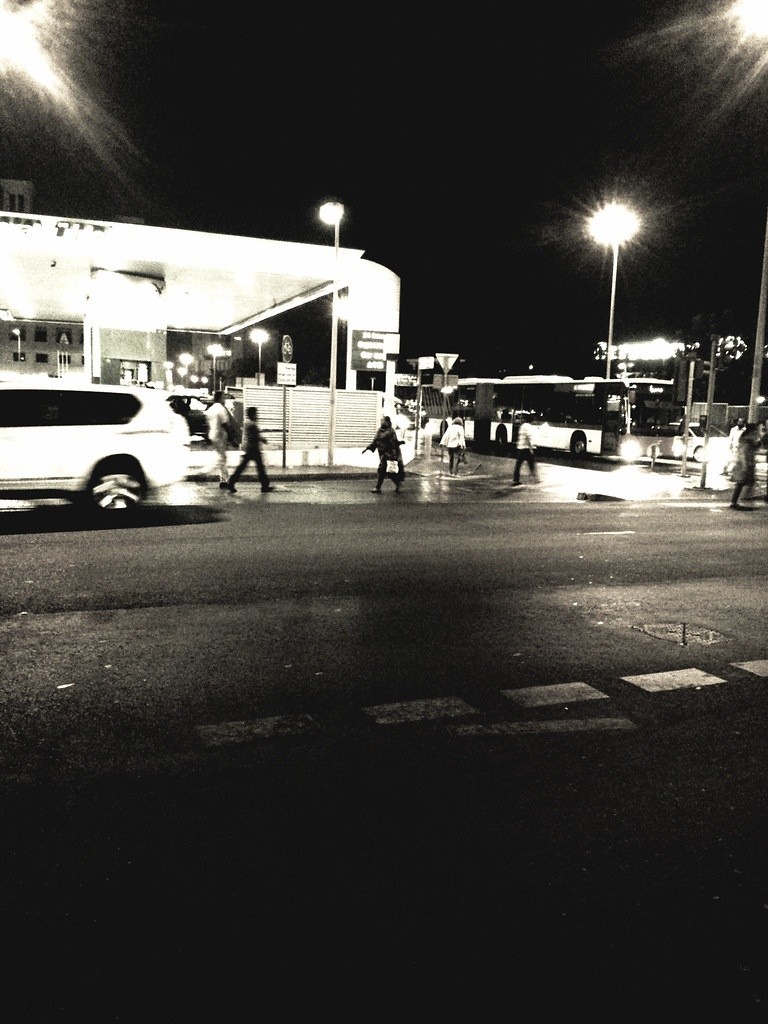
left=0, top=379, right=188, bottom=504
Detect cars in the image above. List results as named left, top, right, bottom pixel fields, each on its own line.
left=182, top=397, right=212, bottom=444
left=671, top=422, right=728, bottom=461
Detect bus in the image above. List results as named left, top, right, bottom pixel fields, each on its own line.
left=420, top=374, right=673, bottom=460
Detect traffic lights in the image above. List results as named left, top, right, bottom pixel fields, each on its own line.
left=715, top=336, right=735, bottom=374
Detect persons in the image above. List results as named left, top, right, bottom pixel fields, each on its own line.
left=360, top=416, right=406, bottom=493
left=206, top=392, right=239, bottom=489
left=511, top=415, right=542, bottom=486
left=727, top=417, right=746, bottom=475
left=728, top=422, right=761, bottom=510
left=439, top=417, right=466, bottom=478
left=222, top=406, right=275, bottom=493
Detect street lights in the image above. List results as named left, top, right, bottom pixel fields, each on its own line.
left=319, top=201, right=344, bottom=470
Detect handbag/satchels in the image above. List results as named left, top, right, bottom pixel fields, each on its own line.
left=386, top=460, right=399, bottom=473
left=458, top=447, right=467, bottom=463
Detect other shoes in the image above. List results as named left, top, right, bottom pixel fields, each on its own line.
left=193, top=475, right=208, bottom=483
left=225, top=483, right=238, bottom=493
left=219, top=482, right=229, bottom=489
left=510, top=482, right=521, bottom=486
left=371, top=488, right=381, bottom=493
left=730, top=502, right=743, bottom=509
left=393, top=487, right=404, bottom=493
left=449, top=474, right=460, bottom=478
left=260, top=486, right=274, bottom=493
